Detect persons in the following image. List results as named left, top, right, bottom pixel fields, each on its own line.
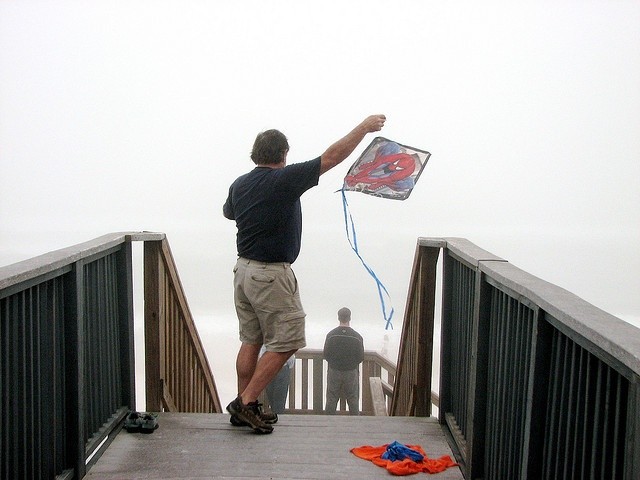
left=323, top=307, right=364, bottom=415
left=258, top=344, right=295, bottom=415
left=223, top=114, right=386, bottom=435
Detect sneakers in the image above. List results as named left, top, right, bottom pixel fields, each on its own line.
left=140, top=411, right=160, bottom=434
left=125, top=411, right=143, bottom=433
left=230, top=402, right=279, bottom=427
left=226, top=395, right=275, bottom=434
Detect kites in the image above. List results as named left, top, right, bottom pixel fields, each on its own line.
left=333, top=136, right=431, bottom=330
